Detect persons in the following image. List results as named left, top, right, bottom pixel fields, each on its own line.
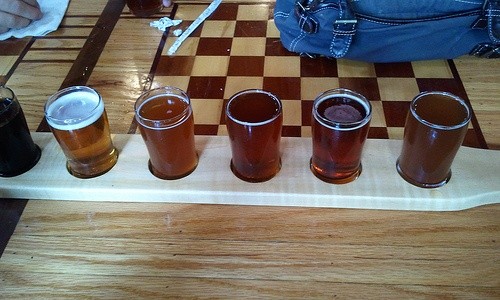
left=0, top=0, right=42, bottom=34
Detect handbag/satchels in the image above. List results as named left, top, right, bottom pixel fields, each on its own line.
left=273, top=0, right=500, bottom=63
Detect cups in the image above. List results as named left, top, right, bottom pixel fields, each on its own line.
left=309, top=88, right=373, bottom=185
left=395, top=90, right=472, bottom=189
left=44, top=85, right=118, bottom=178
left=0, top=86, right=36, bottom=176
left=225, top=88, right=283, bottom=183
left=126, top=0, right=163, bottom=17
left=134, top=85, right=199, bottom=180
